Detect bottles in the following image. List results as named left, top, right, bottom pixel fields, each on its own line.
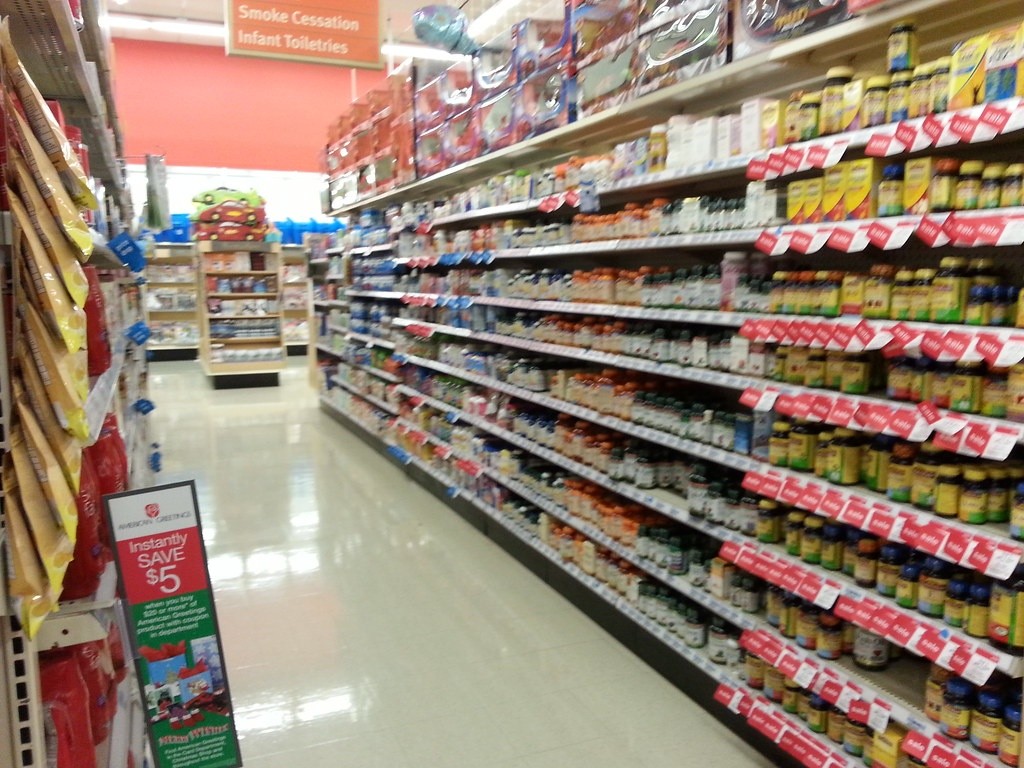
left=311, top=18, right=1024, bottom=768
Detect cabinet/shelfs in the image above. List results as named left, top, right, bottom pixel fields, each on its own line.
left=317, top=0, right=1024, bottom=768
left=144, top=242, right=314, bottom=363
left=0, top=0, right=153, bottom=768
left=195, top=237, right=282, bottom=390
